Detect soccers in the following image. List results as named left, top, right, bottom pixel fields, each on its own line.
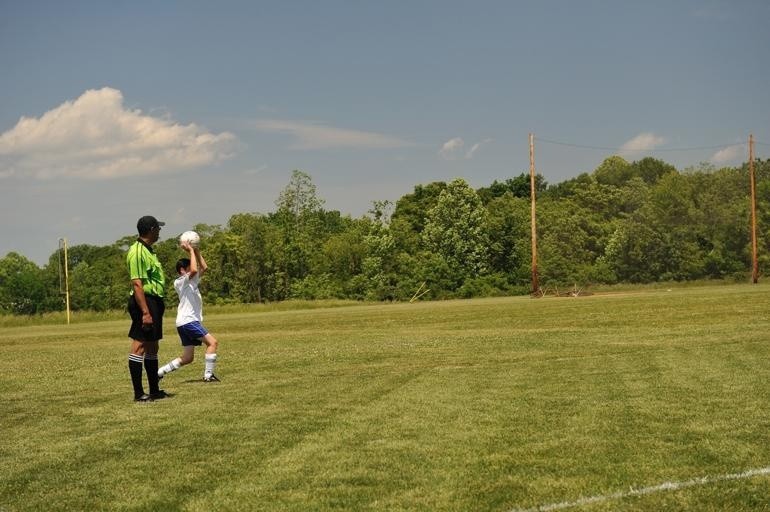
left=179, top=231, right=201, bottom=251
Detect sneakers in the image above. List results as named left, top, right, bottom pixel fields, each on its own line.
left=202, top=373, right=222, bottom=383
left=149, top=390, right=170, bottom=399
left=134, top=393, right=153, bottom=401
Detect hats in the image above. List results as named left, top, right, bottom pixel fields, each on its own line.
left=136, top=215, right=166, bottom=232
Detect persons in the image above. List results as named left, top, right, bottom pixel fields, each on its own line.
left=126, top=216, right=169, bottom=402
left=157, top=240, right=220, bottom=381
